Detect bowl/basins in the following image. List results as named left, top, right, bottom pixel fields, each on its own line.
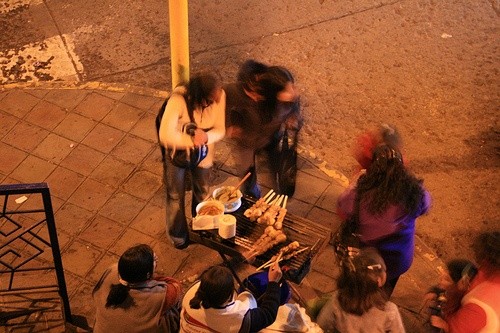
left=213, top=186, right=243, bottom=209
left=195, top=201, right=225, bottom=218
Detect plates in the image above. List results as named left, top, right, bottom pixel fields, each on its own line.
left=211, top=196, right=242, bottom=212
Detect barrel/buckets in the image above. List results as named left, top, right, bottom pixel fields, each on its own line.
left=238, top=271, right=292, bottom=307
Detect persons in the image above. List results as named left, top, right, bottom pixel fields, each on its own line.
left=315, top=249, right=406, bottom=333
left=179, top=251, right=283, bottom=333
left=92, top=244, right=180, bottom=333
left=336, top=143, right=431, bottom=302
left=158, top=65, right=301, bottom=249
left=424, top=231, right=500, bottom=333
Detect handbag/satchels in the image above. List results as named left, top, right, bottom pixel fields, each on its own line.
left=155, top=92, right=209, bottom=169
left=276, top=128, right=297, bottom=199
left=335, top=193, right=360, bottom=266
